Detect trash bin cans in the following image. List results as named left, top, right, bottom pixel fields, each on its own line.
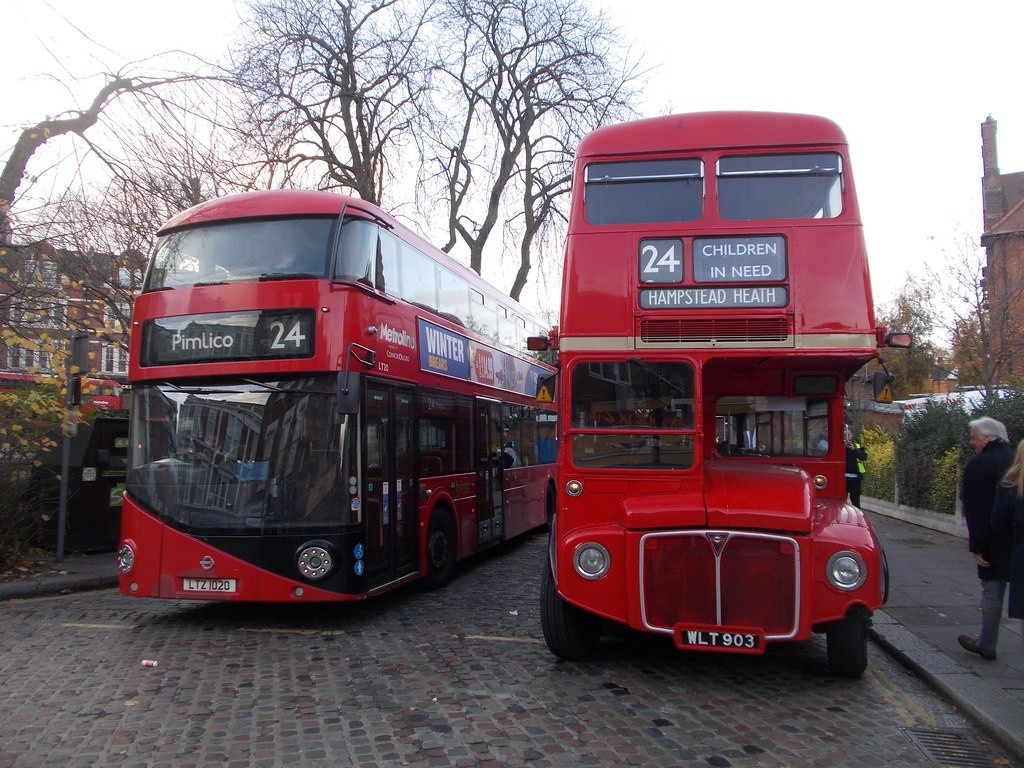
left=70, top=418, right=167, bottom=545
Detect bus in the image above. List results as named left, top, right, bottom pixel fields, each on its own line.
left=116, top=188, right=560, bottom=604
left=0, top=372, right=122, bottom=426
left=525, top=110, right=912, bottom=681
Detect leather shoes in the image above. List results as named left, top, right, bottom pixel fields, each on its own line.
left=958, top=634, right=996, bottom=660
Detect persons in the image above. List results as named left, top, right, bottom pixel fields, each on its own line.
left=504, top=441, right=521, bottom=468
left=957, top=417, right=1024, bottom=660
left=293, top=456, right=348, bottom=522
left=178, top=428, right=223, bottom=476
left=812, top=428, right=868, bottom=510
